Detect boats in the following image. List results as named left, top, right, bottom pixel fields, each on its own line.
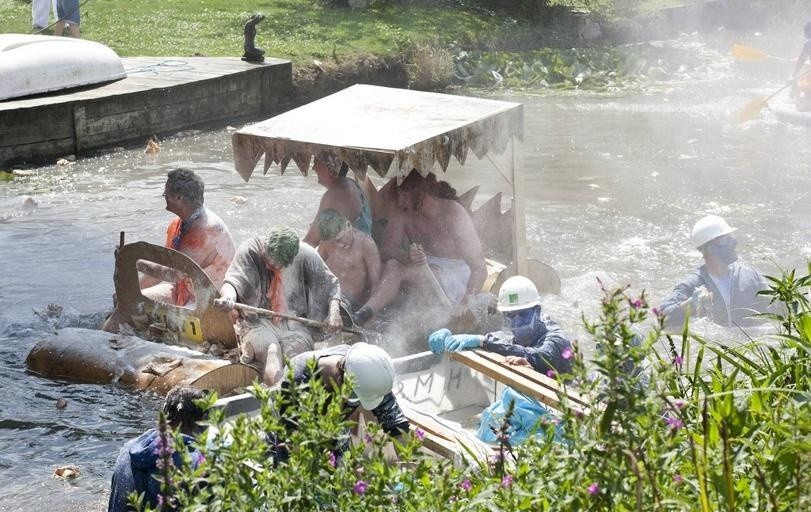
left=767, top=87, right=811, bottom=126
left=25, top=239, right=573, bottom=408
left=208, top=350, right=624, bottom=512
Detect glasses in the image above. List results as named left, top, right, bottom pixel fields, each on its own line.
left=502, top=309, right=538, bottom=335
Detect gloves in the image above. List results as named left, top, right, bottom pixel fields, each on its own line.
left=445, top=334, right=481, bottom=352
left=428, top=327, right=452, bottom=355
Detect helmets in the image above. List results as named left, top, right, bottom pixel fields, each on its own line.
left=496, top=276, right=541, bottom=312
left=345, top=341, right=396, bottom=411
left=691, top=216, right=739, bottom=248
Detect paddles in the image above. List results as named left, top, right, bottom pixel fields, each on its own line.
left=734, top=46, right=801, bottom=63
left=211, top=299, right=380, bottom=336
left=735, top=69, right=810, bottom=120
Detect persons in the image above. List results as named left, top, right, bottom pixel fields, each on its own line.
left=789, top=23, right=811, bottom=117
left=264, top=342, right=417, bottom=466
left=429, top=275, right=574, bottom=382
left=107, top=386, right=222, bottom=512
left=300, top=152, right=485, bottom=329
left=53, top=0, right=80, bottom=38
left=102, top=166, right=232, bottom=334
left=242, top=13, right=265, bottom=61
left=657, top=214, right=787, bottom=331
left=217, top=226, right=343, bottom=391
